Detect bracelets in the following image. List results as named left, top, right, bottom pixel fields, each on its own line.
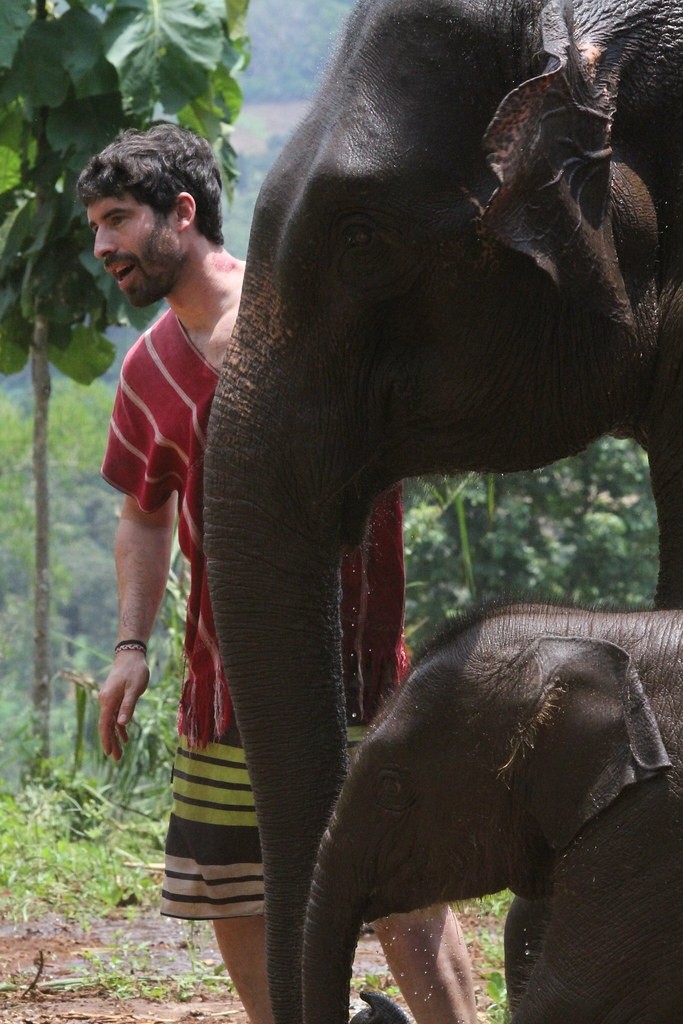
left=114, top=639, right=148, bottom=657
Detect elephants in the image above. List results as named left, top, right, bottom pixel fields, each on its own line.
left=200, top=1, right=683, bottom=1024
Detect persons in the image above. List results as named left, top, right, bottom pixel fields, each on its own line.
left=76, top=126, right=476, bottom=1024
